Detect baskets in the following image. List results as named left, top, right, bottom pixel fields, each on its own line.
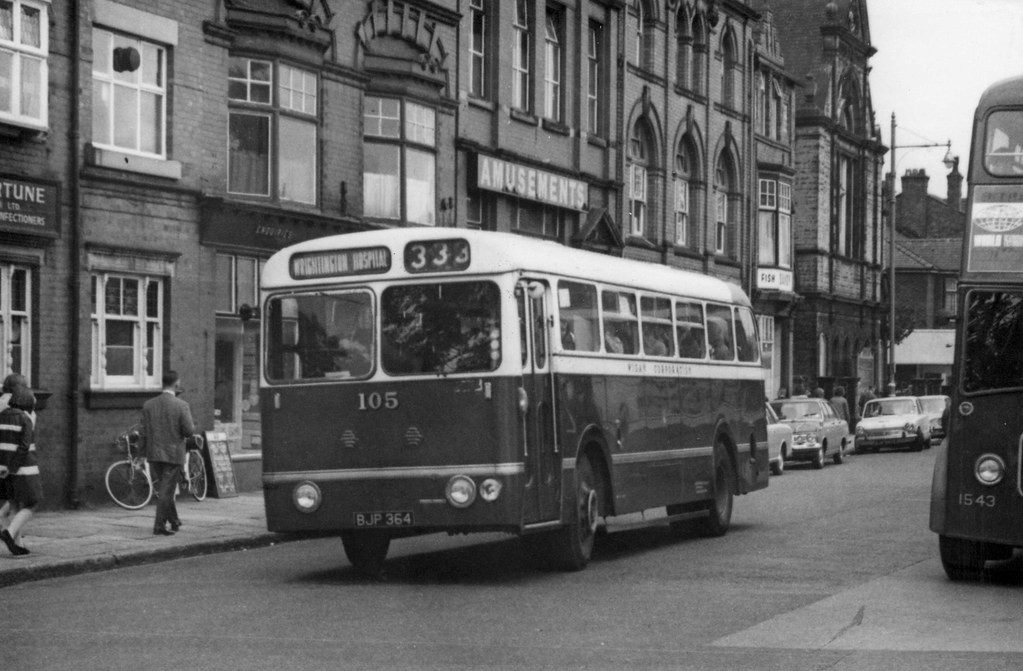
left=116, top=437, right=139, bottom=454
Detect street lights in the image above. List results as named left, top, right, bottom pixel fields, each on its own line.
left=884, top=108, right=957, bottom=397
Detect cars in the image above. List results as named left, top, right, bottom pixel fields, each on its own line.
left=770, top=395, right=851, bottom=470
left=853, top=395, right=931, bottom=455
left=764, top=401, right=794, bottom=475
left=918, top=394, right=950, bottom=439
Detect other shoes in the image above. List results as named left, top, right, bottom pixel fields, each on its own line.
left=153, top=527, right=175, bottom=535
left=0, top=529, right=30, bottom=555
left=172, top=520, right=181, bottom=531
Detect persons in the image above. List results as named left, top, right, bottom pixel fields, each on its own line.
left=415, top=302, right=467, bottom=373
left=830, top=386, right=850, bottom=424
left=136, top=371, right=195, bottom=536
left=777, top=386, right=824, bottom=397
left=0, top=373, right=42, bottom=555
left=604, top=323, right=673, bottom=356
left=859, top=385, right=879, bottom=416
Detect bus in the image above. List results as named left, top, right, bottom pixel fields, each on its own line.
left=251, top=222, right=771, bottom=574
left=928, top=70, right=1023, bottom=584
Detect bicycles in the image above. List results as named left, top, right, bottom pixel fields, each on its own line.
left=105, top=422, right=209, bottom=510
